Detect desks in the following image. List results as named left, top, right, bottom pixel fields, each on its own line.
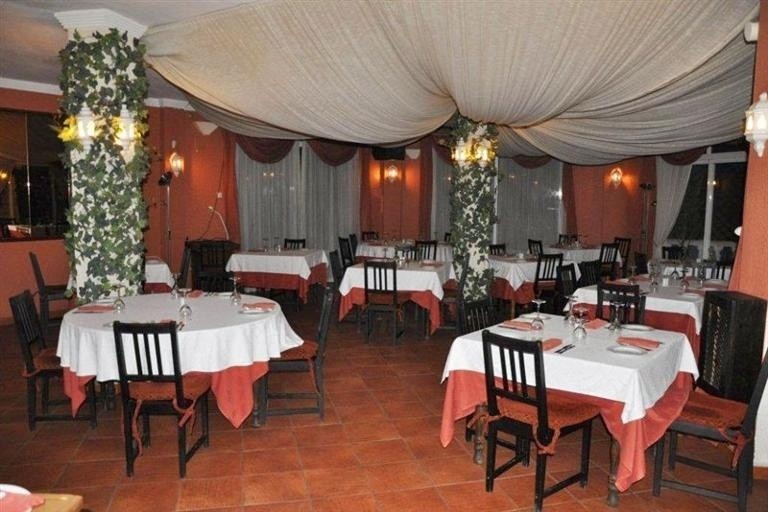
left=441, top=313, right=698, bottom=507
left=60, top=238, right=333, bottom=426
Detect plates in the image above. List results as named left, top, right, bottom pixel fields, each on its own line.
left=241, top=309, right=270, bottom=314
left=1, top=483, right=34, bottom=511
left=608, top=344, right=647, bottom=355
left=520, top=312, right=551, bottom=321
left=619, top=322, right=655, bottom=333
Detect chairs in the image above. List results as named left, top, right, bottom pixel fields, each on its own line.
left=9, top=290, right=106, bottom=428
left=113, top=320, right=212, bottom=478
left=465, top=243, right=737, bottom=461
left=652, top=350, right=768, bottom=512
left=480, top=328, right=598, bottom=511
left=28, top=250, right=77, bottom=331
left=331, top=235, right=634, bottom=345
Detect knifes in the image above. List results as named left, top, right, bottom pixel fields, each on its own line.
left=555, top=342, right=575, bottom=355
left=202, top=290, right=214, bottom=301
left=175, top=322, right=186, bottom=333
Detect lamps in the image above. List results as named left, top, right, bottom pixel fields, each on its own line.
left=115, top=103, right=135, bottom=156
left=169, top=150, right=186, bottom=180
left=384, top=158, right=402, bottom=183
left=74, top=101, right=93, bottom=154
left=609, top=166, right=625, bottom=190
left=743, top=90, right=767, bottom=157
left=158, top=170, right=174, bottom=267
left=453, top=136, right=491, bottom=172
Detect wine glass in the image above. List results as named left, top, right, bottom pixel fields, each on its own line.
left=610, top=302, right=624, bottom=345
left=627, top=264, right=705, bottom=292
left=530, top=300, right=545, bottom=339
left=561, top=234, right=589, bottom=249
left=168, top=272, right=192, bottom=321
left=250, top=238, right=306, bottom=256
left=497, top=248, right=540, bottom=264
left=564, top=295, right=587, bottom=346
left=111, top=285, right=126, bottom=313
left=368, top=235, right=436, bottom=270
left=229, top=275, right=242, bottom=308
